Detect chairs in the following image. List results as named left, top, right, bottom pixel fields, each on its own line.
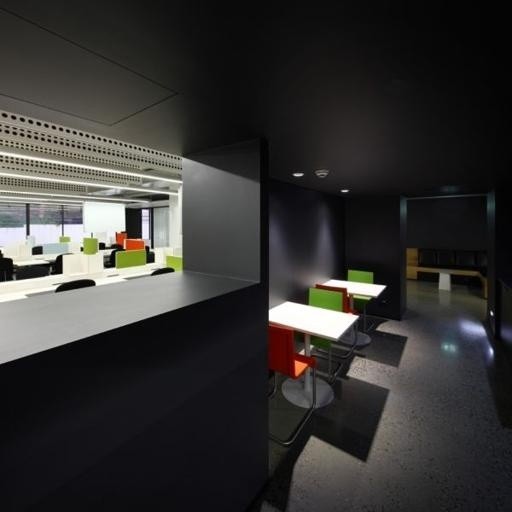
left=0, top=243, right=174, bottom=293
left=268, top=270, right=387, bottom=446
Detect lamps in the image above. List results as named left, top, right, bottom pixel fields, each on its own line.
left=314, top=169, right=329, bottom=179
